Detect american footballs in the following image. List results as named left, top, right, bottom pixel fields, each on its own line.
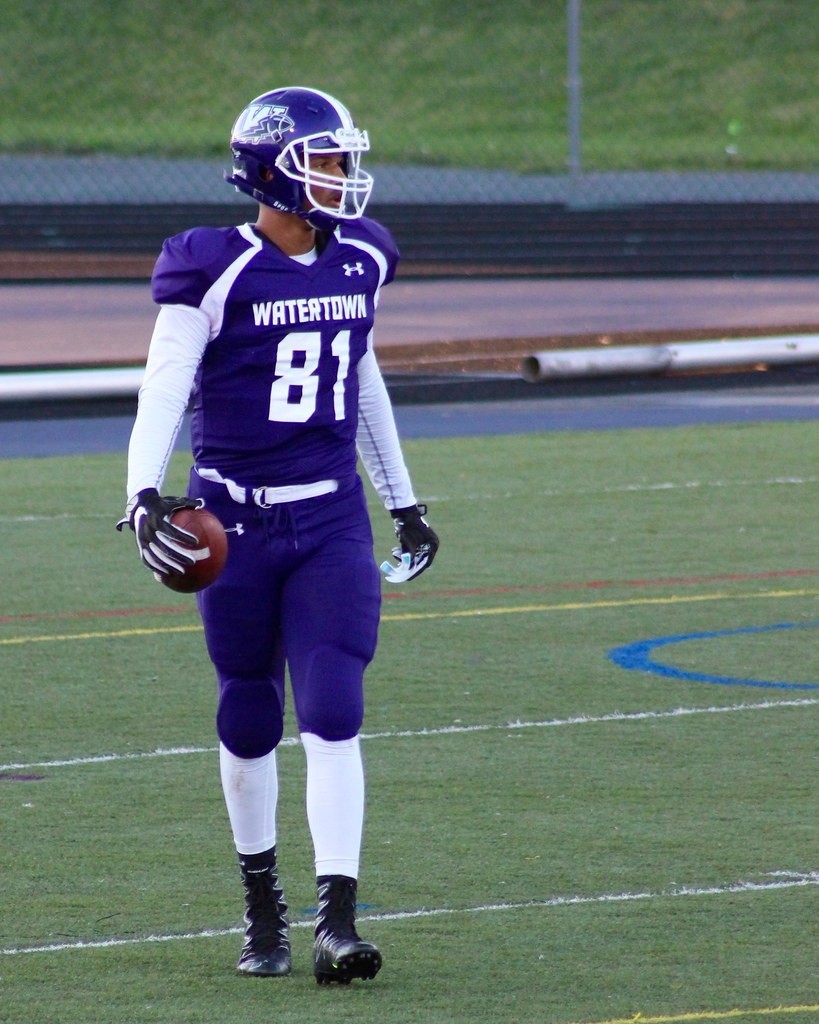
left=158, top=506, right=228, bottom=594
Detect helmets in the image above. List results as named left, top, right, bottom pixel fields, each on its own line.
left=222, top=86, right=374, bottom=232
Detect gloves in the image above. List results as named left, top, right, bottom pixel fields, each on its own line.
left=115, top=488, right=206, bottom=578
left=379, top=504, right=440, bottom=584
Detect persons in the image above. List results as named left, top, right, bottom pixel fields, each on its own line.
left=113, top=87, right=439, bottom=984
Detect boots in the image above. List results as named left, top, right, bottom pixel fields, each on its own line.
left=235, top=845, right=292, bottom=976
left=311, top=875, right=382, bottom=985
